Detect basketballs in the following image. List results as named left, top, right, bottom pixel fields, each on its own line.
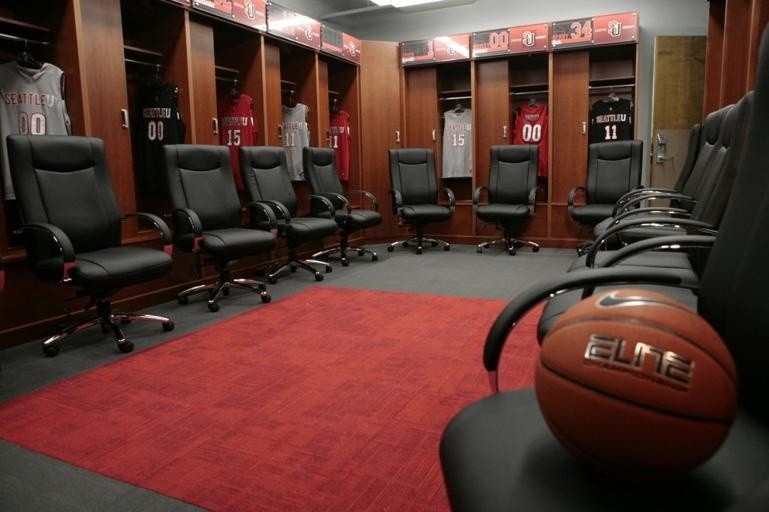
left=534, top=288, right=738, bottom=478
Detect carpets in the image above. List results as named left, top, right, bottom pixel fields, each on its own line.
left=0, top=285, right=546, bottom=512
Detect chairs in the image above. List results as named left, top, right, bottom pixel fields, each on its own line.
left=388, top=148, right=455, bottom=255
left=6, top=133, right=175, bottom=359
left=301, top=146, right=382, bottom=266
left=473, top=144, right=543, bottom=256
left=567, top=140, right=644, bottom=256
left=566, top=104, right=736, bottom=273
left=606, top=124, right=702, bottom=217
left=239, top=145, right=338, bottom=284
left=536, top=91, right=753, bottom=345
left=161, top=143, right=280, bottom=313
left=439, top=23, right=769, bottom=512
left=594, top=105, right=733, bottom=251
